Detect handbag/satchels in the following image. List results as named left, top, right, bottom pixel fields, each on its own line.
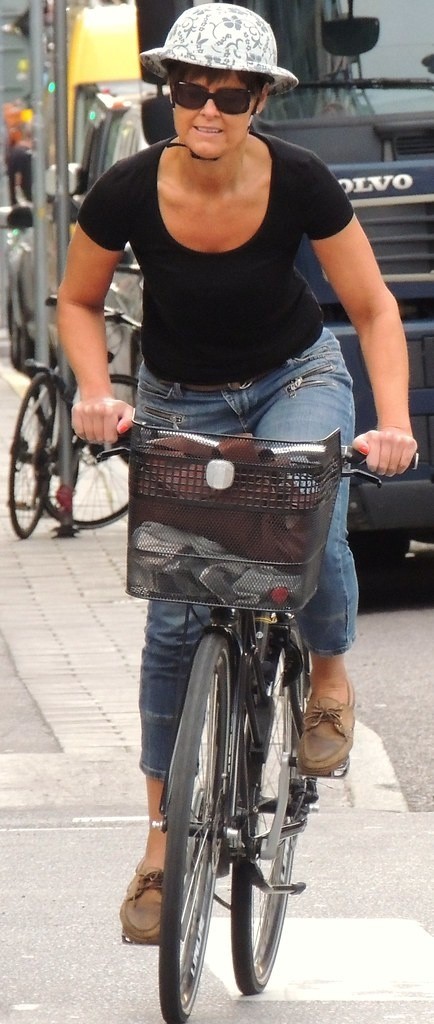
left=129, top=428, right=338, bottom=572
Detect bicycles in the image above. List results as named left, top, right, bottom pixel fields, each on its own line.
left=67, top=418, right=421, bottom=1024
left=5, top=296, right=143, bottom=540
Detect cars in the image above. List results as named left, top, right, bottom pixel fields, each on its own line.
left=1, top=75, right=434, bottom=585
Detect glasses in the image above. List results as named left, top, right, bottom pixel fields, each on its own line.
left=166, top=74, right=255, bottom=115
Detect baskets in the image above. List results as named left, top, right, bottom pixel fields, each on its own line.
left=130, top=409, right=341, bottom=612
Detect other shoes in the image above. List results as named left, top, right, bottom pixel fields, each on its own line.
left=299, top=672, right=355, bottom=774
left=120, top=858, right=163, bottom=943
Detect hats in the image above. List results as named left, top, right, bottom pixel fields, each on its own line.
left=141, top=2, right=299, bottom=96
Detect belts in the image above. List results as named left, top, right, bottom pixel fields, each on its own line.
left=160, top=371, right=268, bottom=391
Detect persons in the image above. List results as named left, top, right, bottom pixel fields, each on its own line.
left=58, top=3, right=417, bottom=944
left=8, top=120, right=54, bottom=207
